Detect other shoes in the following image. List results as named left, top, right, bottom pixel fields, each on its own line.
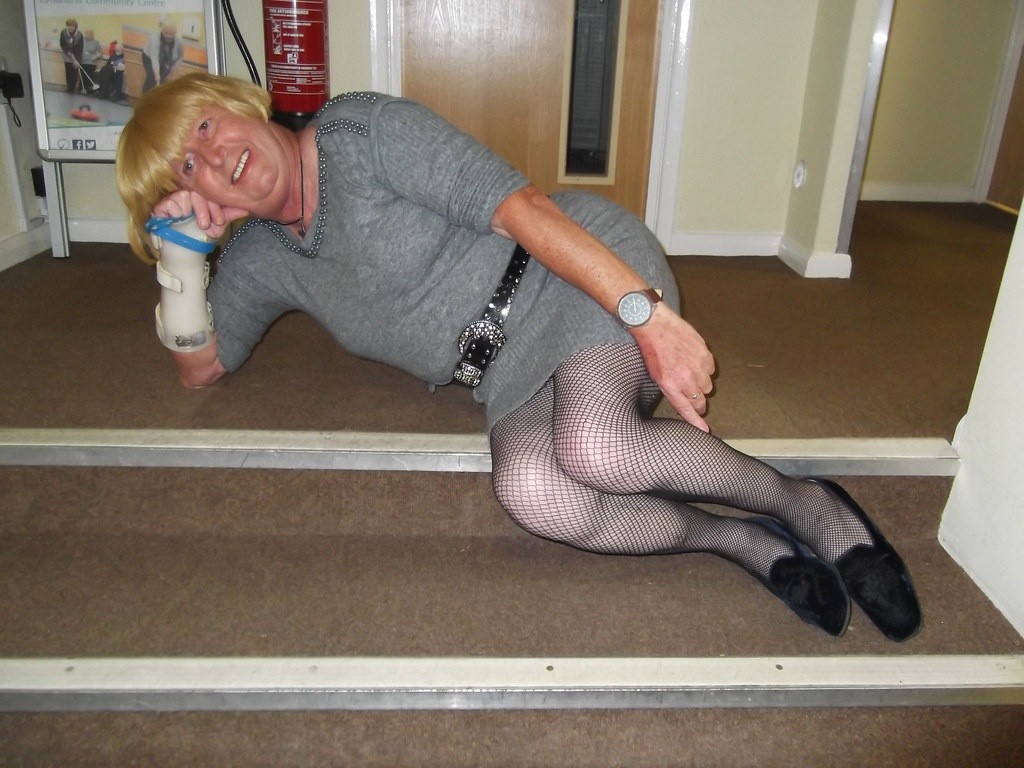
left=784, top=477, right=924, bottom=642
left=743, top=516, right=852, bottom=637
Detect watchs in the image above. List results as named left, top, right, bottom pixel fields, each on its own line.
left=614, top=288, right=662, bottom=330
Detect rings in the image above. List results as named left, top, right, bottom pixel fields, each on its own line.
left=689, top=391, right=702, bottom=399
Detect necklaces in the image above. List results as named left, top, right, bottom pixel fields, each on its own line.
left=273, top=154, right=305, bottom=239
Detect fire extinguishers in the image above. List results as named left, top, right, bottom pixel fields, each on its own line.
left=220, top=0, right=329, bottom=133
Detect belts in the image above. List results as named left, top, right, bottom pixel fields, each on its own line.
left=452, top=244, right=532, bottom=391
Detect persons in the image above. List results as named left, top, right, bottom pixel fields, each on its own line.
left=60, top=18, right=83, bottom=94
left=79, top=29, right=124, bottom=101
left=142, top=21, right=184, bottom=93
left=116, top=73, right=923, bottom=644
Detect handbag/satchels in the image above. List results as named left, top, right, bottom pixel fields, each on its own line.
left=0, top=70, right=25, bottom=127
